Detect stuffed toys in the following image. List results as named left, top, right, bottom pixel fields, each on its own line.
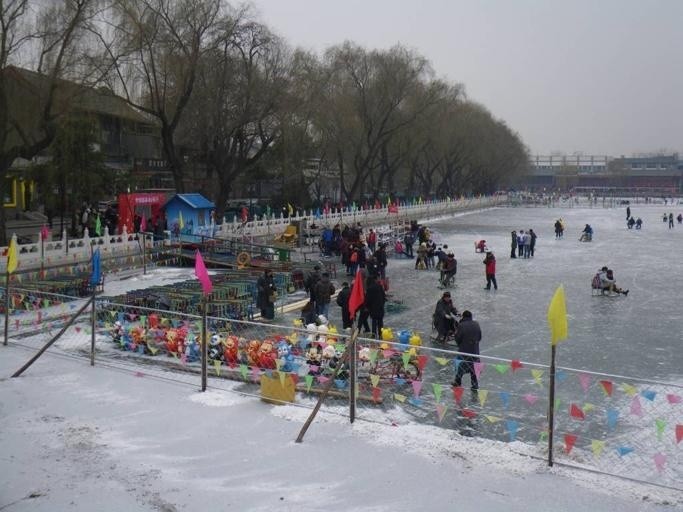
left=107, top=313, right=421, bottom=387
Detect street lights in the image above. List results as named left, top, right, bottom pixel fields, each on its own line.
left=245, top=182, right=256, bottom=221
left=51, top=182, right=73, bottom=241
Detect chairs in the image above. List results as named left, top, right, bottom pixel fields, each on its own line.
left=282, top=225, right=297, bottom=249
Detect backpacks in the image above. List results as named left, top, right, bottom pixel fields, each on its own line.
left=591, top=272, right=603, bottom=290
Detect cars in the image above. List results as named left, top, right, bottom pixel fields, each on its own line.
left=88, top=200, right=119, bottom=221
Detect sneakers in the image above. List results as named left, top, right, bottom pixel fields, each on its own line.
left=483, top=285, right=498, bottom=290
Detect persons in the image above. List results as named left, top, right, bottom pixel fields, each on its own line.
left=591, top=266, right=628, bottom=296
left=555, top=216, right=563, bottom=239
left=578, top=223, right=592, bottom=241
left=134, top=215, right=165, bottom=235
left=73, top=198, right=117, bottom=238
left=625, top=207, right=643, bottom=231
left=662, top=212, right=682, bottom=228
left=510, top=228, right=538, bottom=257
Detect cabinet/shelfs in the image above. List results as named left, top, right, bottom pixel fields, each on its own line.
left=375, top=217, right=411, bottom=254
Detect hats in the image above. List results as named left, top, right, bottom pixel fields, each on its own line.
left=443, top=292, right=451, bottom=298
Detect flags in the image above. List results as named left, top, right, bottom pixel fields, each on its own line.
left=42, top=225, right=49, bottom=240
left=139, top=207, right=149, bottom=234
left=194, top=248, right=213, bottom=295
left=89, top=248, right=101, bottom=288
left=7, top=237, right=20, bottom=272
left=547, top=285, right=568, bottom=347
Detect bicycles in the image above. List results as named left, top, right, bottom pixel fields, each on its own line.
left=364, top=347, right=419, bottom=381
